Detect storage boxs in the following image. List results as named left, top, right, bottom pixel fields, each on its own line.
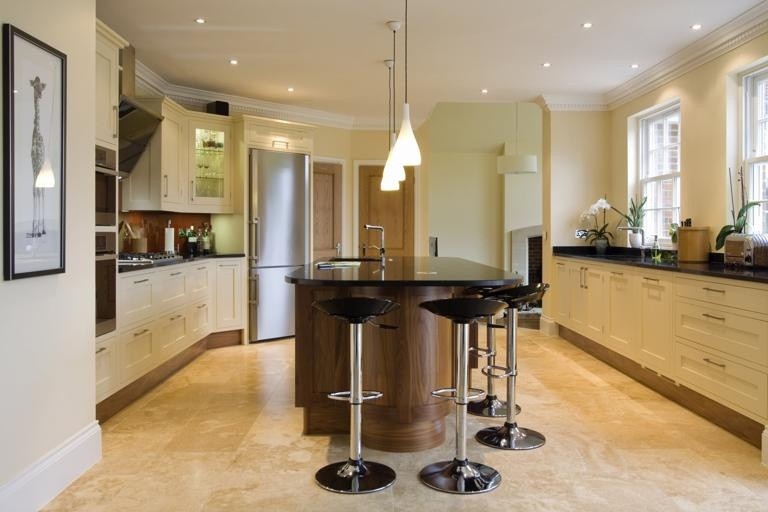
left=677, top=225, right=712, bottom=263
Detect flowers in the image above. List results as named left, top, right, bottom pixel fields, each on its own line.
left=668, top=223, right=678, bottom=243
left=578, top=194, right=614, bottom=247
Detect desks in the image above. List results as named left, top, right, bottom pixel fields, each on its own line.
left=285, top=253, right=527, bottom=453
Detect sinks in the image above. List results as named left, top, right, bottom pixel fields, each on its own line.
left=328, top=257, right=381, bottom=262
left=588, top=254, right=640, bottom=261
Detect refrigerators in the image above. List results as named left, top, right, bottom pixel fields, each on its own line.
left=249, top=146, right=313, bottom=345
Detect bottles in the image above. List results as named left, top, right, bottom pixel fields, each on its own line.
left=202, top=229, right=210, bottom=256
left=164, top=227, right=174, bottom=252
left=651, top=235, right=660, bottom=255
left=187, top=225, right=197, bottom=257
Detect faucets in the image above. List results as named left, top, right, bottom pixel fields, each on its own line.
left=370, top=261, right=385, bottom=281
left=617, top=226, right=646, bottom=258
left=363, top=224, right=385, bottom=261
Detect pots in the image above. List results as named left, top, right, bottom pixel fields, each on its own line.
left=128, top=237, right=148, bottom=254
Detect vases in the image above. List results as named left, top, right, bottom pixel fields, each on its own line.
left=595, top=239, right=608, bottom=256
left=672, top=242, right=679, bottom=251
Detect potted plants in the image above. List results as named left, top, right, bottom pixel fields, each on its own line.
left=611, top=194, right=647, bottom=250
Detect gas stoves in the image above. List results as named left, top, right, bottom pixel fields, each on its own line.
left=118, top=251, right=183, bottom=264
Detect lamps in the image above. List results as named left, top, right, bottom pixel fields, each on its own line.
left=34, top=60, right=55, bottom=189
left=379, top=0, right=422, bottom=194
left=497, top=104, right=537, bottom=175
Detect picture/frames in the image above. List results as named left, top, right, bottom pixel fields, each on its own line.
left=4, top=23, right=66, bottom=282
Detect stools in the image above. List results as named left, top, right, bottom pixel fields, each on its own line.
left=310, top=297, right=401, bottom=495
left=418, top=282, right=549, bottom=494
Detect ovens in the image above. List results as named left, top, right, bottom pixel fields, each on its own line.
left=96, top=164, right=116, bottom=338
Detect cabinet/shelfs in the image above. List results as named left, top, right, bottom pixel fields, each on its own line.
left=553, top=254, right=768, bottom=450
